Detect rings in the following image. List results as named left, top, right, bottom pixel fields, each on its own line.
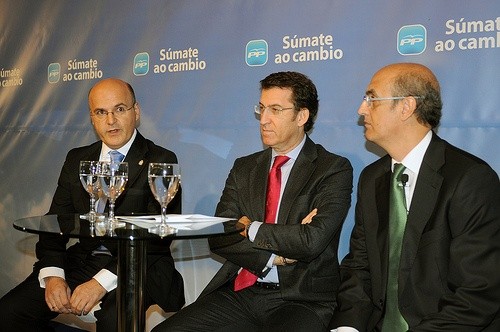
left=52, top=305, right=56, bottom=308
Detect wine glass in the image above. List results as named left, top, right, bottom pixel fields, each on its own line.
left=94, top=162, right=129, bottom=228
left=148, top=162, right=182, bottom=235
left=80, top=160, right=104, bottom=219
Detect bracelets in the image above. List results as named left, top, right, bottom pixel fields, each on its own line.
left=282, top=257, right=288, bottom=264
left=247, top=222, right=252, bottom=237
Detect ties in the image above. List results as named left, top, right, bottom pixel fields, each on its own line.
left=381, top=164, right=409, bottom=332
left=234, top=155, right=291, bottom=292
left=96, top=151, right=125, bottom=212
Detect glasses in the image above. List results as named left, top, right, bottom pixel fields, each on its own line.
left=254, top=105, right=298, bottom=115
left=89, top=104, right=134, bottom=118
left=361, top=95, right=425, bottom=108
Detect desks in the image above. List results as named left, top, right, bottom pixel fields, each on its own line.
left=12, top=214, right=245, bottom=332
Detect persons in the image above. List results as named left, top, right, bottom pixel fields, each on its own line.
left=0, top=77, right=186, bottom=331
left=326, top=63, right=500, bottom=332
left=151, top=72, right=355, bottom=332
